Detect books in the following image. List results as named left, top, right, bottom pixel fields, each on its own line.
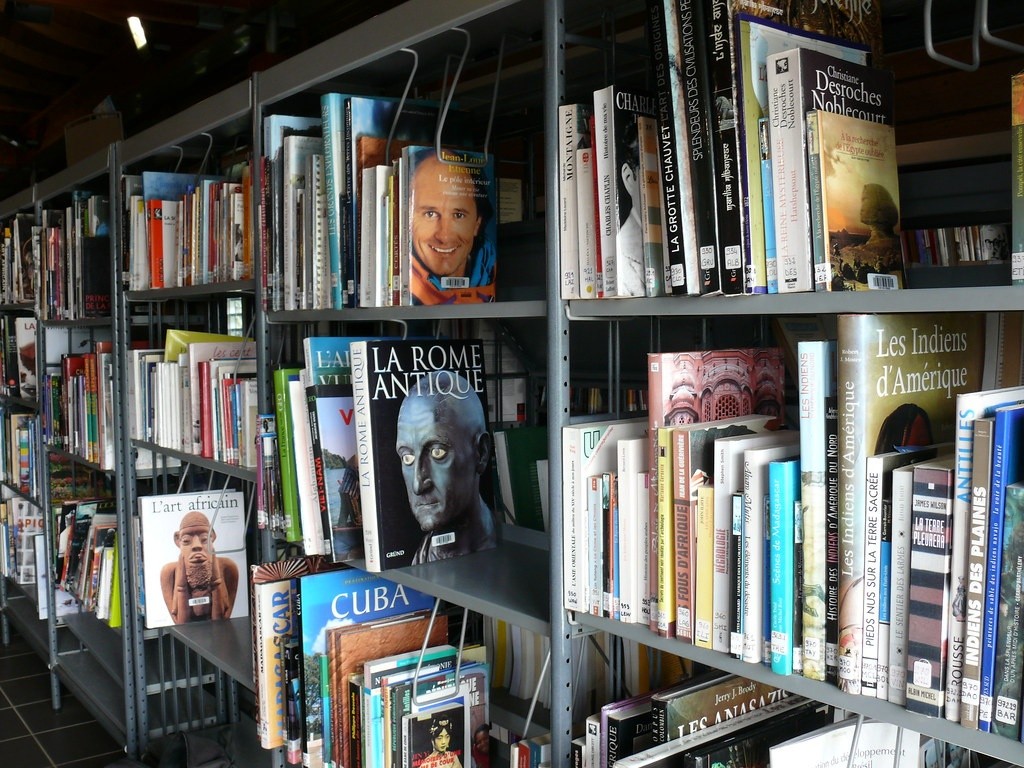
left=559, top=1, right=1024, bottom=300
left=563, top=310, right=1024, bottom=745
left=0, top=92, right=553, bottom=768
left=570, top=669, right=1024, bottom=768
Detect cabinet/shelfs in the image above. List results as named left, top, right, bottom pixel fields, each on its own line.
left=1, top=0, right=1024, bottom=768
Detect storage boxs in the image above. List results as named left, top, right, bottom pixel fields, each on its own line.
left=63, top=110, right=123, bottom=168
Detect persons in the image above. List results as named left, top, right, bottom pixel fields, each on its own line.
left=472, top=724, right=490, bottom=768
left=409, top=149, right=497, bottom=306
left=419, top=717, right=463, bottom=768
